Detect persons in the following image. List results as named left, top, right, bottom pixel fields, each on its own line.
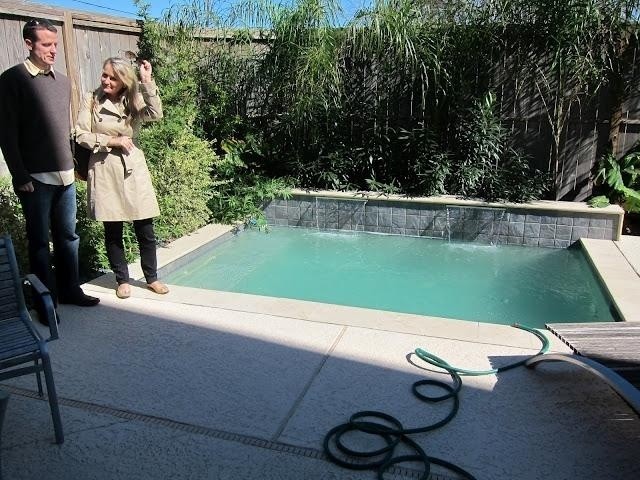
left=0, top=15, right=101, bottom=326
left=72, top=56, right=170, bottom=298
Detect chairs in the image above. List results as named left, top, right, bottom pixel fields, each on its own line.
left=524, top=320, right=640, bottom=431
left=0, top=232, right=66, bottom=447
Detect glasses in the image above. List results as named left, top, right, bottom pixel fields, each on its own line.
left=27, top=18, right=53, bottom=25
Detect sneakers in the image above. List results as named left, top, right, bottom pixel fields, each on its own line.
left=147, top=280, right=168, bottom=294
left=59, top=290, right=101, bottom=307
left=117, top=283, right=130, bottom=299
left=37, top=308, right=60, bottom=326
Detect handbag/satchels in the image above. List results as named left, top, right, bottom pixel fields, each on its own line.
left=71, top=130, right=91, bottom=178
